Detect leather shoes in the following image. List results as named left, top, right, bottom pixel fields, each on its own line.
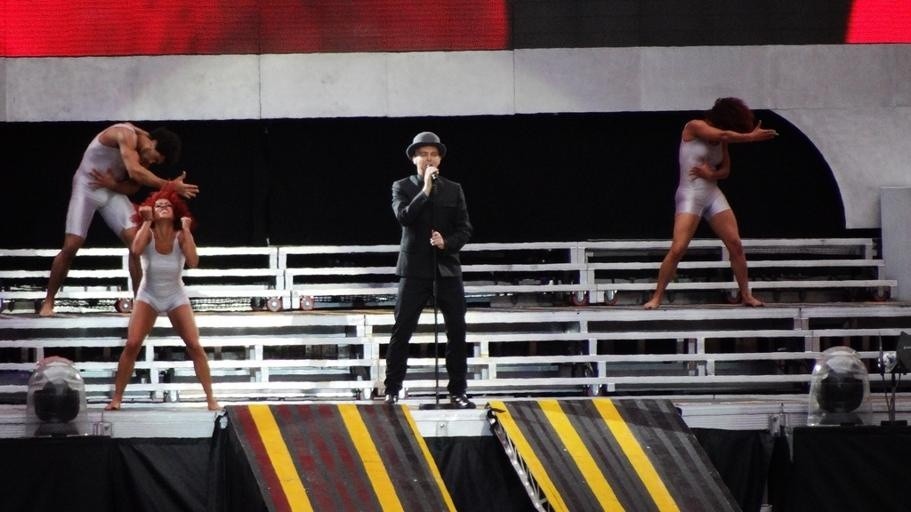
left=451, top=393, right=476, bottom=408
left=384, top=393, right=398, bottom=404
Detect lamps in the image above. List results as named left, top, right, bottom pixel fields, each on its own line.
left=30, top=356, right=86, bottom=437
left=808, top=346, right=870, bottom=426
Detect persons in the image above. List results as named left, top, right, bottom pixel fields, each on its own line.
left=39, top=123, right=200, bottom=317
left=385, top=131, right=476, bottom=409
left=104, top=191, right=223, bottom=410
left=643, top=97, right=779, bottom=309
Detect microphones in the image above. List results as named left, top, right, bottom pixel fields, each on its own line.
left=427, top=164, right=439, bottom=185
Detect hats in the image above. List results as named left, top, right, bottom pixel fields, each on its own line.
left=406, top=132, right=446, bottom=159
